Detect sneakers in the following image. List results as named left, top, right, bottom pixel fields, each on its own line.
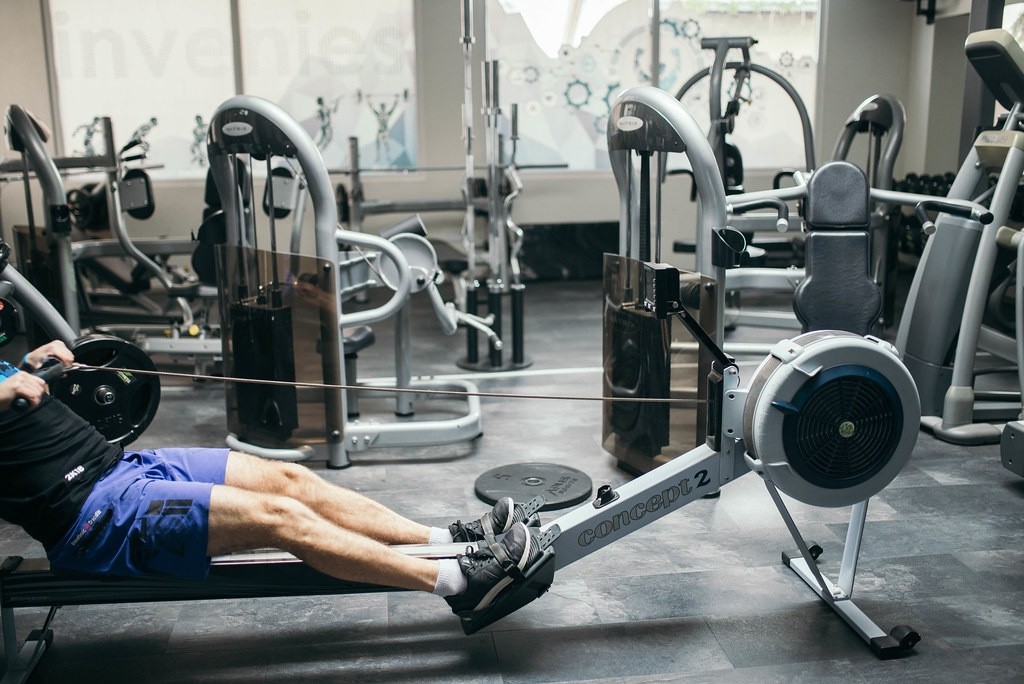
left=429, top=498, right=514, bottom=559
left=442, top=522, right=531, bottom=620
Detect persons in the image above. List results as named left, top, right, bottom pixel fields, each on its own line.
left=0, top=338, right=531, bottom=623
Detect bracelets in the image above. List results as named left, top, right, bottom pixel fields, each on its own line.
left=23, top=353, right=31, bottom=365
